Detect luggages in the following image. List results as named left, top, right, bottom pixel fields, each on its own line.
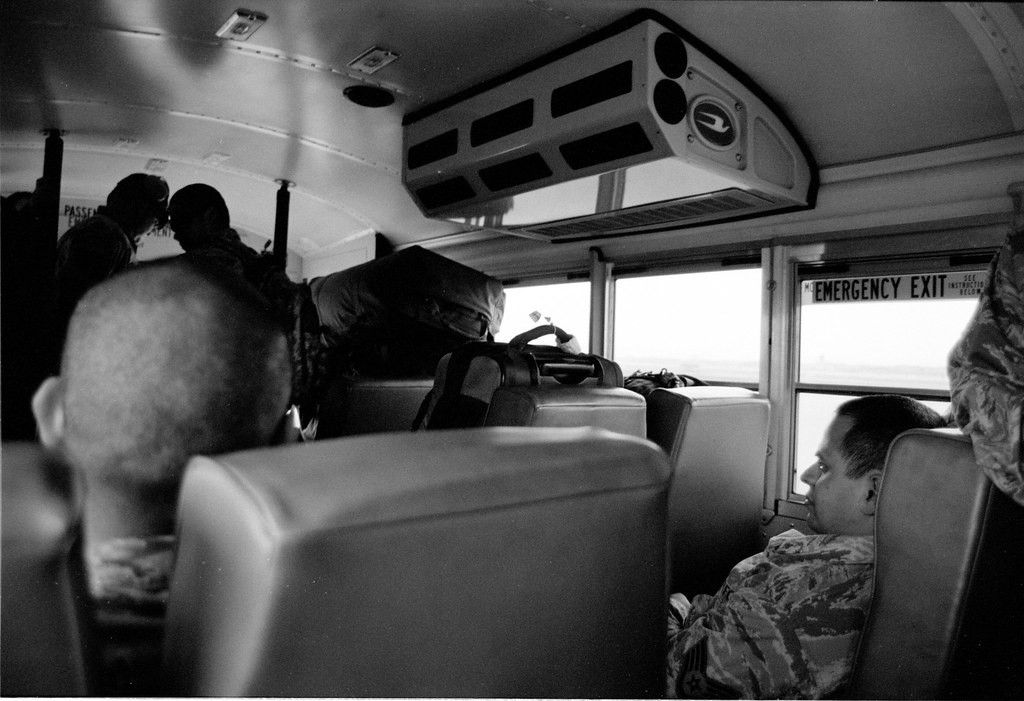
left=408, top=326, right=623, bottom=432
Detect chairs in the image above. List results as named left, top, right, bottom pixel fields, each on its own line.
left=0, top=378, right=1024, bottom=699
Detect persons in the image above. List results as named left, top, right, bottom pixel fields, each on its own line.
left=32, top=263, right=298, bottom=697
left=949, top=201, right=1023, bottom=511
left=666, top=395, right=938, bottom=699
left=1, top=127, right=293, bottom=441
